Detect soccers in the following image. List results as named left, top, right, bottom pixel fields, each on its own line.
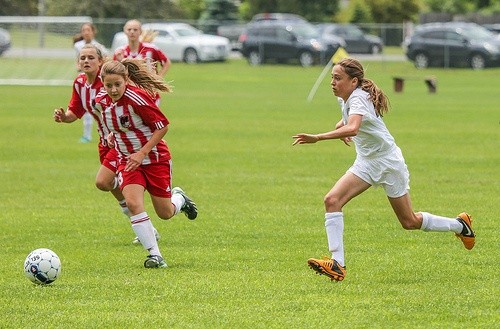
left=23, top=248, right=61, bottom=285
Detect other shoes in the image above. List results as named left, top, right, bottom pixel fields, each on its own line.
left=78, top=136, right=92, bottom=143
left=132, top=227, right=158, bottom=244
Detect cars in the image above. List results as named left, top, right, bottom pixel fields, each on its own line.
left=405, top=21, right=500, bottom=70
left=111, top=23, right=247, bottom=64
left=314, top=22, right=384, bottom=54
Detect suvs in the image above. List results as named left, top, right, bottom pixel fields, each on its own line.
left=238, top=12, right=347, bottom=66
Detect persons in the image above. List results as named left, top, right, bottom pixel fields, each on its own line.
left=87, top=60, right=197, bottom=267
left=52, top=45, right=159, bottom=244
left=114, top=19, right=169, bottom=109
left=73, top=22, right=109, bottom=143
left=293, top=59, right=475, bottom=281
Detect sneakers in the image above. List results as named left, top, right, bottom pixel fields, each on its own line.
left=144, top=254, right=167, bottom=268
left=454, top=212, right=476, bottom=250
left=307, top=256, right=346, bottom=282
left=171, top=187, right=198, bottom=220
